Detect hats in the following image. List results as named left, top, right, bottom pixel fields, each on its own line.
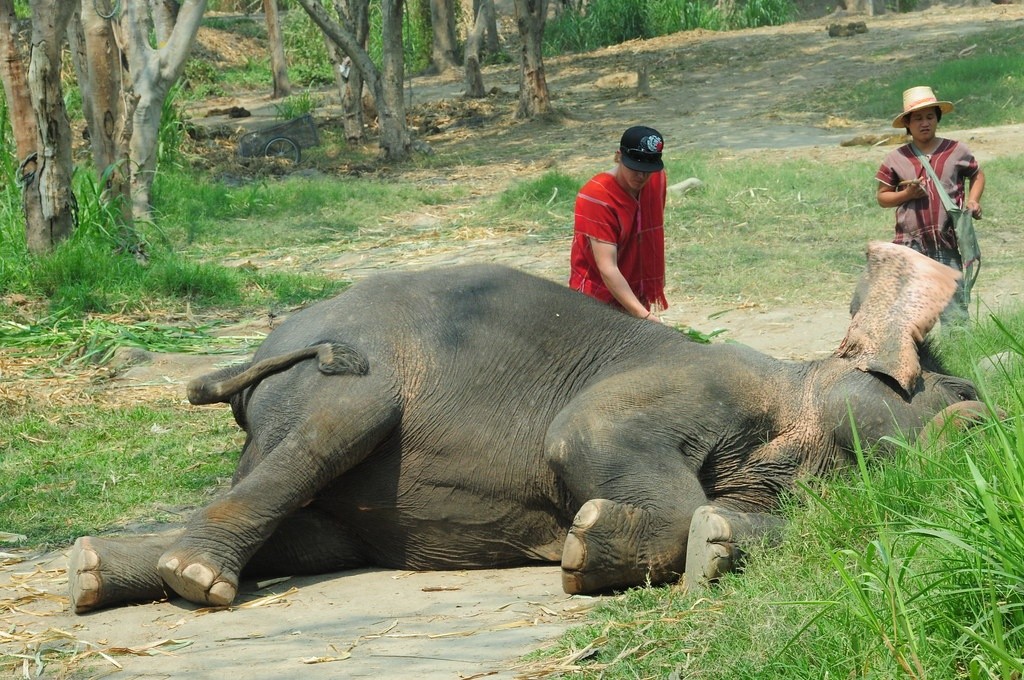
left=620, top=126, right=665, bottom=172
left=892, top=86, right=955, bottom=128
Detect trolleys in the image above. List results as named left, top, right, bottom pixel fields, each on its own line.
left=240, top=113, right=338, bottom=176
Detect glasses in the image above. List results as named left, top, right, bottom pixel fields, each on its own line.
left=622, top=147, right=663, bottom=163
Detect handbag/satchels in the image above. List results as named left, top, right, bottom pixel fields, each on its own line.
left=949, top=206, right=982, bottom=307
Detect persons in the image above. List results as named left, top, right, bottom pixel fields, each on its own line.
left=568, top=125, right=669, bottom=325
left=876, top=86, right=986, bottom=323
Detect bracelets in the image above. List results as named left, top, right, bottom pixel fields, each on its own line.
left=645, top=311, right=651, bottom=319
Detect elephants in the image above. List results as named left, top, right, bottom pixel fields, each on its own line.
left=64, top=237, right=1011, bottom=618
